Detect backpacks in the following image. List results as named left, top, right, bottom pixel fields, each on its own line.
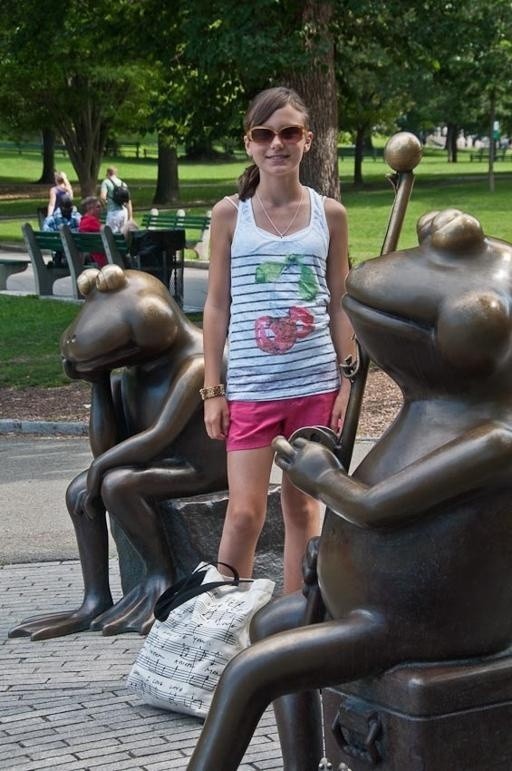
left=56, top=186, right=73, bottom=214
left=107, top=177, right=129, bottom=205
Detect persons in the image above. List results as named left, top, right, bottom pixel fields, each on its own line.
left=199, top=82, right=360, bottom=599
left=45, top=167, right=135, bottom=273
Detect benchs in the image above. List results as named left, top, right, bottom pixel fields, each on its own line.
left=140, top=206, right=213, bottom=260
left=1, top=252, right=33, bottom=293
left=23, top=221, right=190, bottom=314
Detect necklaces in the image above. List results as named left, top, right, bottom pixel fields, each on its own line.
left=254, top=188, right=307, bottom=236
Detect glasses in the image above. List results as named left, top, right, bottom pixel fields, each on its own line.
left=246, top=124, right=306, bottom=146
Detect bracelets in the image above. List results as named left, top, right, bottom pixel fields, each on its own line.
left=199, top=383, right=225, bottom=401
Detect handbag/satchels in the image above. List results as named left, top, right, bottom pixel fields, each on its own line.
left=125, top=560, right=276, bottom=720
